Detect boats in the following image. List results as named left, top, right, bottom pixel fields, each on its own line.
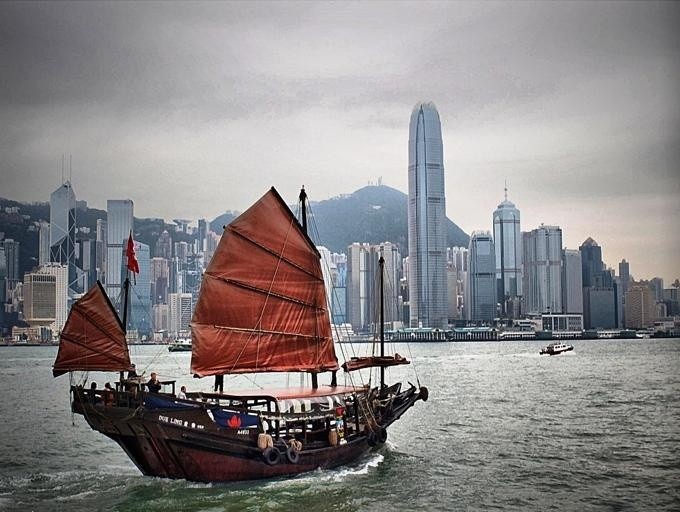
left=167, top=336, right=192, bottom=352
left=539, top=339, right=575, bottom=356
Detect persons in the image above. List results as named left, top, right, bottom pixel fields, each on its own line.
left=146, top=372, right=162, bottom=394
left=333, top=401, right=346, bottom=434
left=127, top=363, right=142, bottom=378
left=99, top=382, right=117, bottom=408
left=177, top=385, right=188, bottom=400
left=87, top=382, right=105, bottom=406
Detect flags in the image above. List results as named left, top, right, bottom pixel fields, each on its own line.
left=211, top=408, right=262, bottom=431
left=125, top=233, right=141, bottom=275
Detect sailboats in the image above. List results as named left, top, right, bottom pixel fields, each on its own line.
left=46, top=181, right=434, bottom=482
